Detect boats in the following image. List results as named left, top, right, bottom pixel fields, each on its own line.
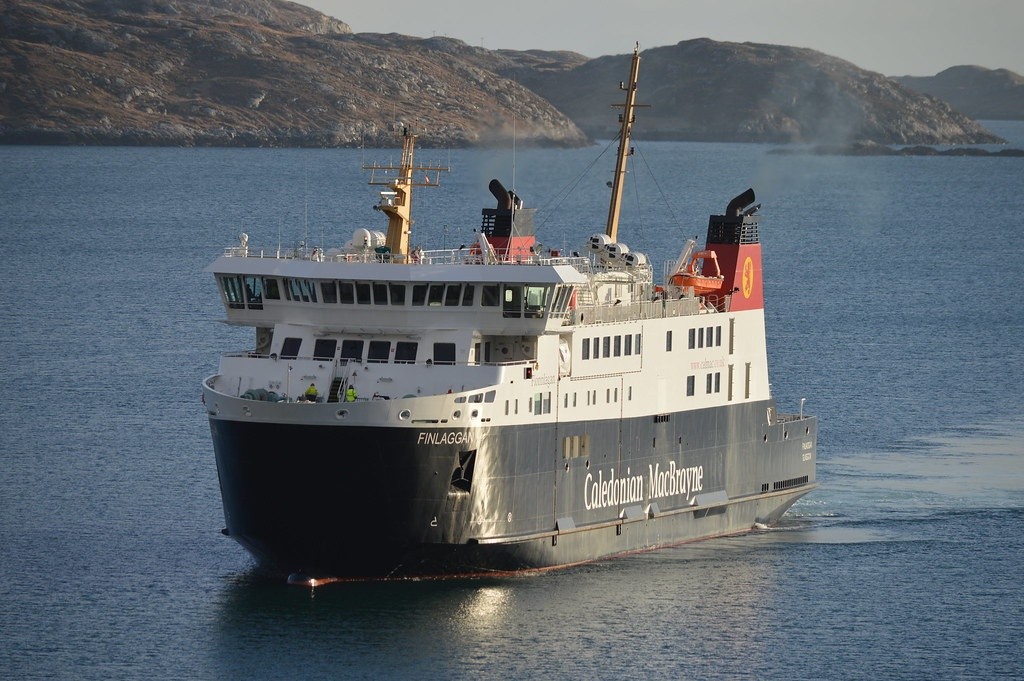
left=199, top=39, right=819, bottom=587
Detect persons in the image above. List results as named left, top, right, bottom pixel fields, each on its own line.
left=345, top=384, right=358, bottom=403
left=244, top=283, right=255, bottom=309
left=410, top=245, right=422, bottom=265
left=304, top=383, right=319, bottom=404
